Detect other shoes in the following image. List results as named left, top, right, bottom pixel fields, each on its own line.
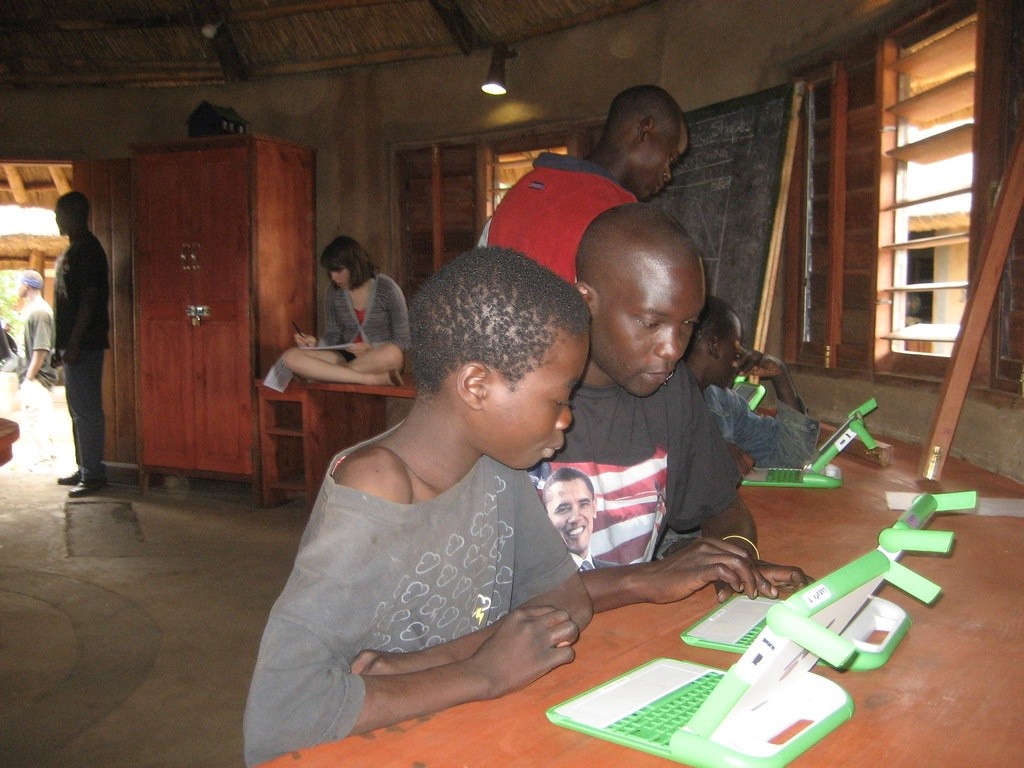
left=389, top=368, right=404, bottom=386
left=67, top=482, right=100, bottom=497
left=58, top=470, right=82, bottom=485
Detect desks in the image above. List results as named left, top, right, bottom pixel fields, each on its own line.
left=244, top=407, right=1024, bottom=768
left=255, top=374, right=416, bottom=509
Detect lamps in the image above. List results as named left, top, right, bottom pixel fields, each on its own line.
left=481, top=42, right=518, bottom=96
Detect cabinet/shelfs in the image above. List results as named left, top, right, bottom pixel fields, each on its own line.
left=128, top=132, right=320, bottom=507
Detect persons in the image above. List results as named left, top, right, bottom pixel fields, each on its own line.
left=52, top=191, right=110, bottom=497
left=0, top=269, right=57, bottom=473
left=488, top=84, right=689, bottom=284
left=683, top=296, right=820, bottom=477
left=283, top=236, right=411, bottom=386
left=241, top=245, right=593, bottom=768
left=526, top=202, right=816, bottom=613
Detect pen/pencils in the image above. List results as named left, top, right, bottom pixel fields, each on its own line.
left=290, top=319, right=309, bottom=348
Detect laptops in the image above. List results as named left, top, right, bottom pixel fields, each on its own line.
left=543, top=377, right=980, bottom=768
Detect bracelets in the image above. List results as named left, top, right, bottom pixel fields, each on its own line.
left=723, top=536, right=759, bottom=560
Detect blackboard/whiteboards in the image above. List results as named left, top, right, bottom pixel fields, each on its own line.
left=639, top=79, right=805, bottom=353
